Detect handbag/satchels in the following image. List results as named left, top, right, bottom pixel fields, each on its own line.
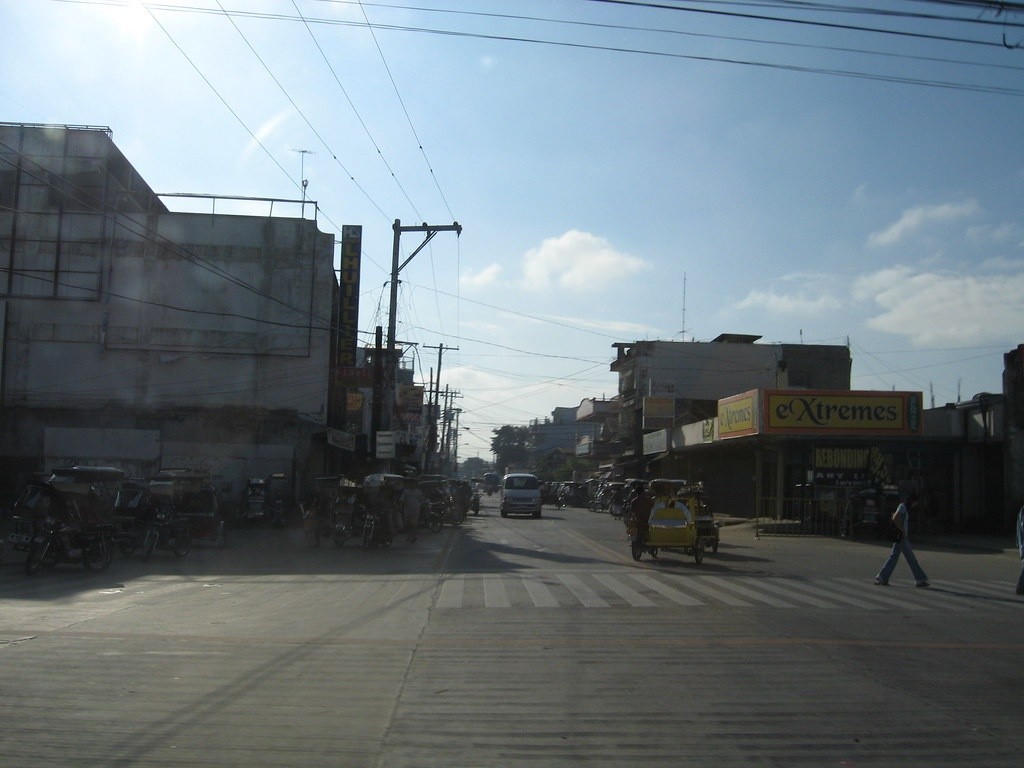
left=885, top=510, right=906, bottom=544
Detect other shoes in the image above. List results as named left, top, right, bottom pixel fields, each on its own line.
left=916, top=582, right=930, bottom=588
left=873, top=576, right=890, bottom=586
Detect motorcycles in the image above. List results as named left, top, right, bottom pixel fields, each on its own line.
left=538, top=477, right=723, bottom=564
left=1, top=462, right=497, bottom=575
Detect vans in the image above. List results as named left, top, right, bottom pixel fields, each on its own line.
left=497, top=473, right=543, bottom=519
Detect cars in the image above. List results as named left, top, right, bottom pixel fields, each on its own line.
left=843, top=482, right=904, bottom=538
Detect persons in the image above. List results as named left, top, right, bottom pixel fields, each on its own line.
left=876, top=490, right=930, bottom=586
left=1014, top=504, right=1024, bottom=595
left=400, top=479, right=647, bottom=547
